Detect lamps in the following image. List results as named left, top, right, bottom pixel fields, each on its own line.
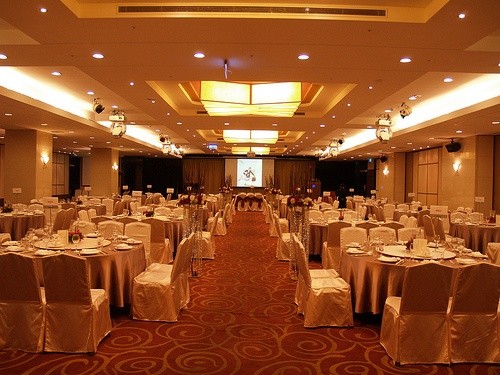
left=223, top=129, right=279, bottom=144
left=92, top=98, right=105, bottom=115
left=109, top=112, right=128, bottom=139
left=200, top=80, right=302, bottom=118
left=329, top=139, right=340, bottom=156
left=338, top=135, right=345, bottom=144
left=232, top=147, right=270, bottom=155
left=399, top=101, right=411, bottom=119
left=445, top=139, right=461, bottom=152
left=376, top=114, right=394, bottom=143
left=162, top=134, right=172, bottom=154
left=380, top=155, right=387, bottom=162
left=159, top=133, right=165, bottom=142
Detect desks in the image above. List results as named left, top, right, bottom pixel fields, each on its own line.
left=0, top=239, right=145, bottom=307
left=449, top=221, right=500, bottom=252
left=338, top=245, right=492, bottom=314
left=0, top=212, right=44, bottom=240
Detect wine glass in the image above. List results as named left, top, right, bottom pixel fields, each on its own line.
left=123, top=208, right=129, bottom=216
left=374, top=241, right=386, bottom=258
left=434, top=234, right=466, bottom=261
left=42, top=234, right=50, bottom=250
left=485, top=216, right=490, bottom=225
left=71, top=234, right=80, bottom=253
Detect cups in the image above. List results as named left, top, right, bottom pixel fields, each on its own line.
left=456, top=219, right=463, bottom=224
left=368, top=213, right=376, bottom=222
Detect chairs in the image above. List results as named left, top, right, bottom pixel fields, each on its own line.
left=0, top=191, right=235, bottom=353
left=264, top=194, right=500, bottom=363
left=237, top=192, right=262, bottom=212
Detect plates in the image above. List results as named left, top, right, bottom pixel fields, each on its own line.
left=378, top=257, right=397, bottom=262
left=2, top=240, right=54, bottom=255
left=114, top=246, right=132, bottom=250
left=126, top=241, right=142, bottom=244
left=81, top=251, right=101, bottom=255
left=462, top=259, right=474, bottom=264
left=346, top=244, right=364, bottom=254
